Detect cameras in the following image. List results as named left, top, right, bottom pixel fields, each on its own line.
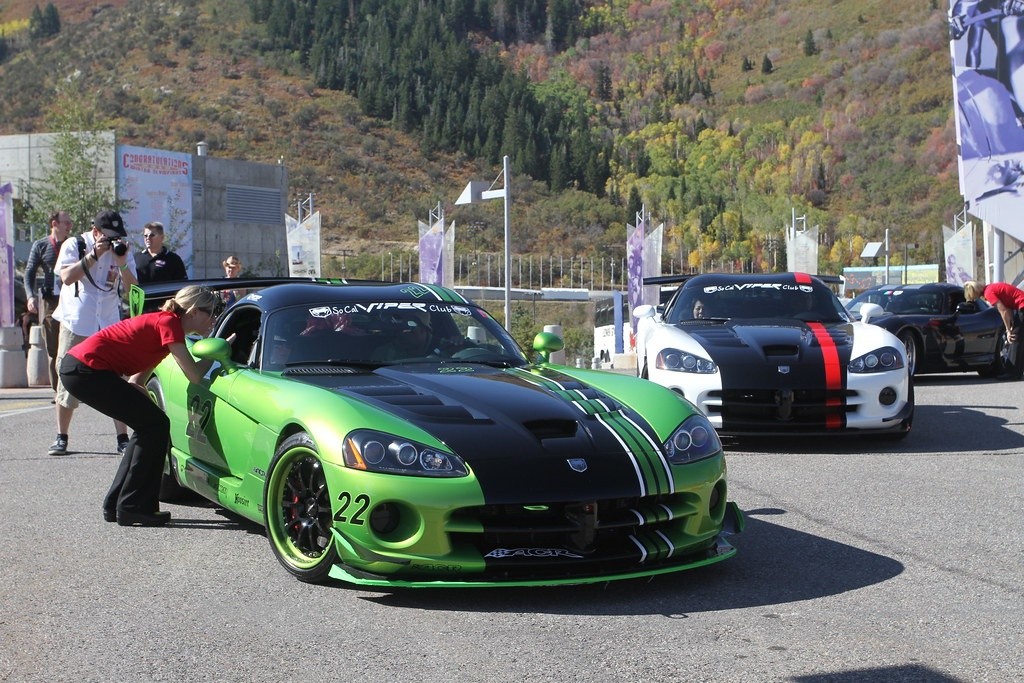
left=104, top=237, right=127, bottom=256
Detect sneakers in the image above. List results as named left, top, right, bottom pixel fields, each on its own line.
left=48, top=437, right=67, bottom=454
left=117, top=445, right=126, bottom=459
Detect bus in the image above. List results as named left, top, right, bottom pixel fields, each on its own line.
left=593, top=285, right=679, bottom=370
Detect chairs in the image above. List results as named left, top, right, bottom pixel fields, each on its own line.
left=256, top=311, right=319, bottom=373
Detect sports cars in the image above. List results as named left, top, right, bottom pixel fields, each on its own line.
left=844, top=282, right=1011, bottom=378
left=632, top=274, right=914, bottom=440
left=142, top=279, right=744, bottom=588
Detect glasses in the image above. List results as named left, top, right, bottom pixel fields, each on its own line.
left=144, top=233, right=161, bottom=238
left=199, top=304, right=215, bottom=318
left=390, top=314, right=419, bottom=330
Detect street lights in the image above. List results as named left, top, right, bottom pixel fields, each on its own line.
left=860, top=228, right=889, bottom=285
left=454, top=154, right=511, bottom=336
left=905, top=242, right=919, bottom=284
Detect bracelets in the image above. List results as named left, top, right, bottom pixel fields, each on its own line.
left=119, top=264, right=127, bottom=271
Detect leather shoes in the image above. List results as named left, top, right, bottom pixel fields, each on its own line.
left=102, top=508, right=117, bottom=522
left=117, top=509, right=171, bottom=526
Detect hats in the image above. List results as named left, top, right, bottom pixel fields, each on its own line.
left=94, top=210, right=127, bottom=238
left=389, top=306, right=434, bottom=331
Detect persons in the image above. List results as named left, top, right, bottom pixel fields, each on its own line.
left=207, top=256, right=247, bottom=307
left=47, top=209, right=139, bottom=457
left=921, top=294, right=950, bottom=313
left=688, top=297, right=712, bottom=319
left=778, top=294, right=816, bottom=318
left=133, top=222, right=189, bottom=286
left=24, top=211, right=72, bottom=404
left=59, top=285, right=235, bottom=526
left=964, top=280, right=1024, bottom=379
left=248, top=333, right=291, bottom=367
left=370, top=307, right=467, bottom=360
